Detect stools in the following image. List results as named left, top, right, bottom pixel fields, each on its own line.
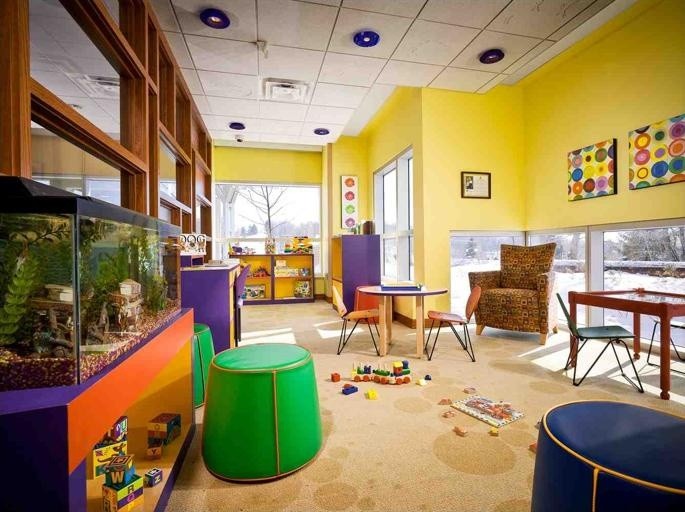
left=201, top=342, right=322, bottom=482
left=531, top=402, right=685, bottom=512
left=193, top=322, right=215, bottom=409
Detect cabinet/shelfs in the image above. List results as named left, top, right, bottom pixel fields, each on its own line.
left=32, top=133, right=121, bottom=178
left=230, top=254, right=315, bottom=305
left=180, top=260, right=241, bottom=357
left=330, top=234, right=380, bottom=314
left=0, top=305, right=196, bottom=512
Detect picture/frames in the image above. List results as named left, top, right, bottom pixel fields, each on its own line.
left=461, top=172, right=491, bottom=199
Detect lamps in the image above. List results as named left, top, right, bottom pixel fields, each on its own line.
left=353, top=31, right=380, bottom=47
left=229, top=123, right=244, bottom=130
left=480, top=49, right=504, bottom=64
left=200, top=8, right=230, bottom=29
left=315, top=129, right=329, bottom=136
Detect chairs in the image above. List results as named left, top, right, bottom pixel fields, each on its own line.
left=424, top=286, right=481, bottom=362
left=468, top=242, right=556, bottom=345
left=647, top=316, right=685, bottom=374
left=556, top=293, right=644, bottom=393
left=332, top=285, right=380, bottom=357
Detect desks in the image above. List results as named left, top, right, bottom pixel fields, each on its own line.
left=568, top=287, right=685, bottom=400
left=356, top=284, right=448, bottom=359
